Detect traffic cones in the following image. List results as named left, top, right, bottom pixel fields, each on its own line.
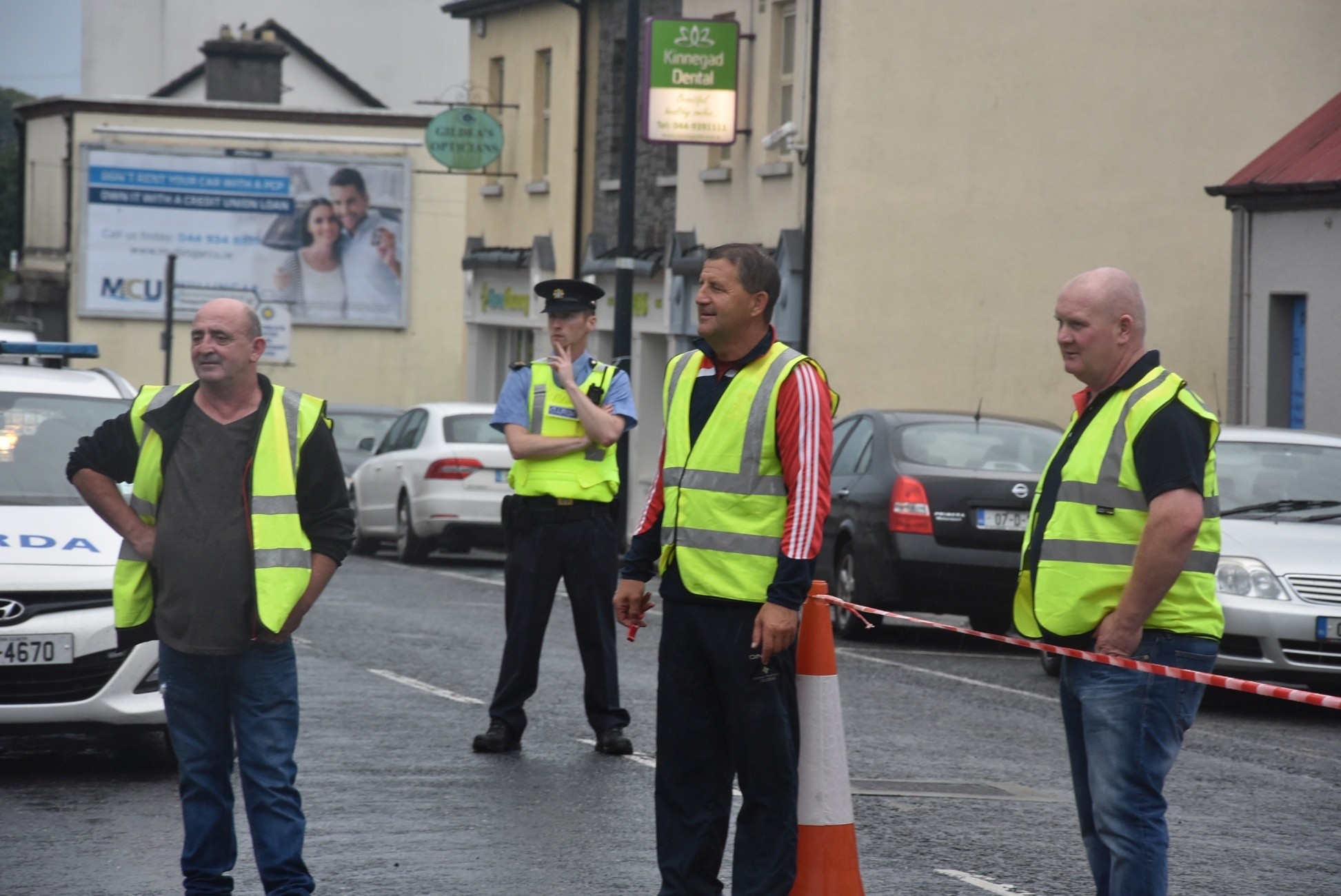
left=784, top=580, right=867, bottom=896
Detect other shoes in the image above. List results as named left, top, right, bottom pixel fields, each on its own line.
left=593, top=730, right=634, bottom=755
left=473, top=722, right=523, bottom=751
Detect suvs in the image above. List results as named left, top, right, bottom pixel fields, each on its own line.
left=0, top=326, right=176, bottom=761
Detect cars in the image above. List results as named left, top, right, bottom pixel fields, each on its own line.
left=821, top=405, right=1067, bottom=645
left=343, top=403, right=518, bottom=567
left=1211, top=422, right=1341, bottom=722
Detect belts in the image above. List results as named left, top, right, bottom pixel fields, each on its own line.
left=523, top=495, right=594, bottom=507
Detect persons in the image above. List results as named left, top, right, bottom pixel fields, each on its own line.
left=275, top=168, right=401, bottom=318
left=610, top=241, right=838, bottom=895
left=66, top=299, right=355, bottom=895
left=1011, top=267, right=1223, bottom=894
left=472, top=280, right=636, bottom=755
left=271, top=198, right=346, bottom=319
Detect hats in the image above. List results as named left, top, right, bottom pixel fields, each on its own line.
left=534, top=279, right=605, bottom=314
